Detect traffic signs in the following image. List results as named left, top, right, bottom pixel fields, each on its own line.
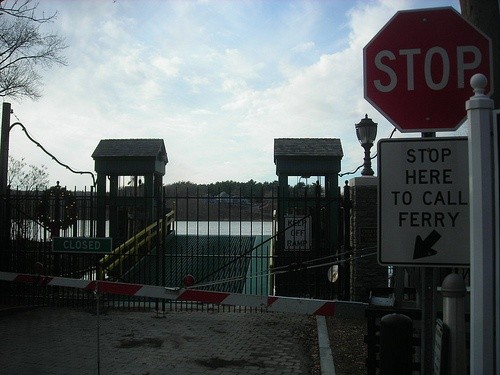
left=375, top=136, right=473, bottom=270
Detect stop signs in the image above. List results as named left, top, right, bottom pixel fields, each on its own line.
left=360, top=4, right=495, bottom=135
